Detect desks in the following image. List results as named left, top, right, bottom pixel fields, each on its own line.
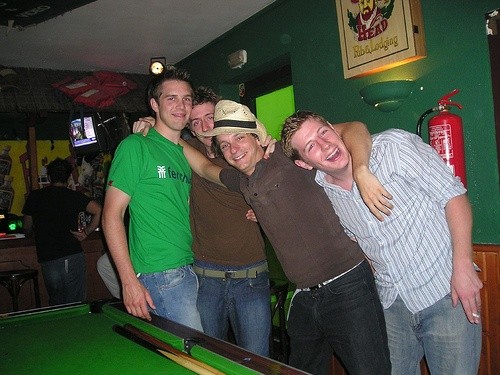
left=0, top=296, right=313, bottom=375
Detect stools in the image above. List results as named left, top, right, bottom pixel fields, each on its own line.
left=0, top=269, right=41, bottom=312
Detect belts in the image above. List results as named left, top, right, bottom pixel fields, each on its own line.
left=193, top=260, right=268, bottom=279
left=286, top=258, right=367, bottom=321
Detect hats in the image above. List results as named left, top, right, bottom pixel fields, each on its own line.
left=194, top=100, right=267, bottom=143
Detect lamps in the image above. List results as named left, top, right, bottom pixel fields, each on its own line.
left=149, top=57, right=168, bottom=77
left=359, top=80, right=413, bottom=111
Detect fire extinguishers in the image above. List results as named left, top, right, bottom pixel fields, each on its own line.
left=417, top=88, right=468, bottom=193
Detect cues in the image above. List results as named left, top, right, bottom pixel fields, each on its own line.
left=111, top=323, right=226, bottom=375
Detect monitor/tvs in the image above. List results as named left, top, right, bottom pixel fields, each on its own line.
left=67, top=115, right=104, bottom=155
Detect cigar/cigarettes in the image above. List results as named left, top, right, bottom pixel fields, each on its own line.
left=472, top=314, right=480, bottom=318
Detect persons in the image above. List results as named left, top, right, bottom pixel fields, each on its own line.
left=280, top=110, right=483, bottom=375
left=96, top=252, right=122, bottom=300
left=183, top=86, right=277, bottom=358
left=100, top=68, right=203, bottom=336
left=20, top=157, right=102, bottom=305
left=131, top=101, right=394, bottom=375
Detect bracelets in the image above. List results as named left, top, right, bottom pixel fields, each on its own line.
left=84, top=230, right=87, bottom=235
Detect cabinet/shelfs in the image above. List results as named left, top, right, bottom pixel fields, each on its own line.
left=0, top=235, right=112, bottom=314
